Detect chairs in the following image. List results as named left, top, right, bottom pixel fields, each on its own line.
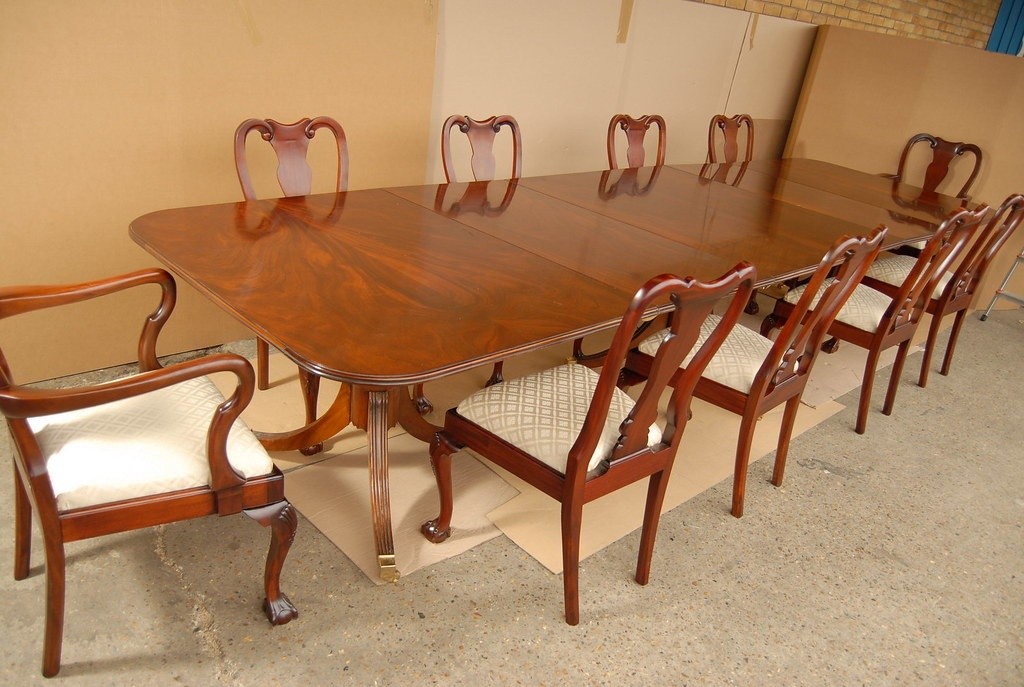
left=0, top=116, right=1024, bottom=680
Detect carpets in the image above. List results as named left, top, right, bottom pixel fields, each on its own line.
left=204, top=294, right=959, bottom=586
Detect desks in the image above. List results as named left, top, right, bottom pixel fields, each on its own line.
left=129, top=157, right=990, bottom=583
